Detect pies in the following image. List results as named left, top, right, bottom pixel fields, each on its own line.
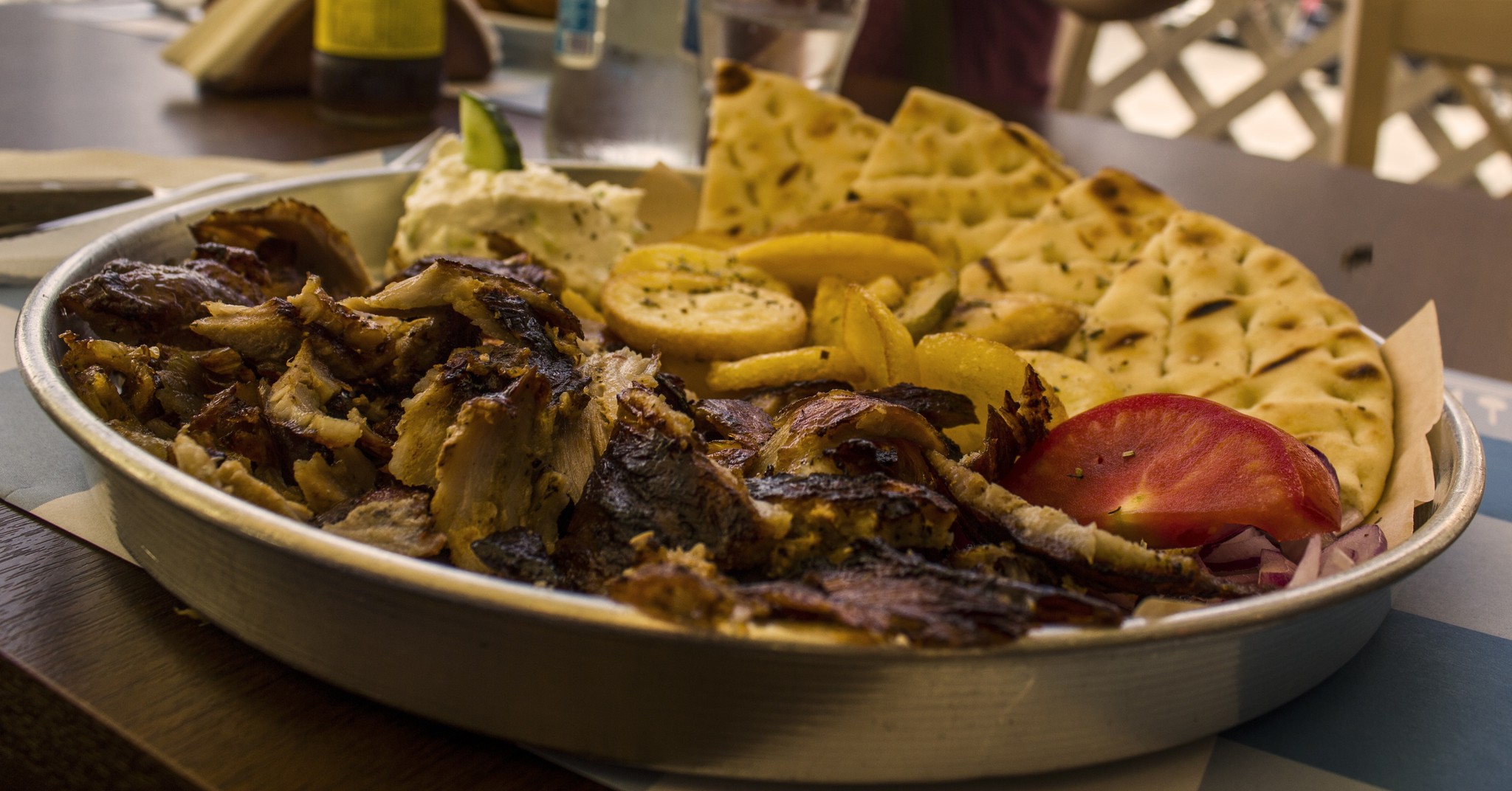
left=678, top=58, right=1396, bottom=555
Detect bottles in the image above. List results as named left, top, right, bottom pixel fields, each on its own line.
left=546, top=2, right=707, bottom=167
left=308, top=0, right=447, bottom=132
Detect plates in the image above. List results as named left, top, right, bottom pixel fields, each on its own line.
left=15, top=167, right=1485, bottom=789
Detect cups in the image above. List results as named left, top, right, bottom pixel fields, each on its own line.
left=703, top=0, right=865, bottom=96
